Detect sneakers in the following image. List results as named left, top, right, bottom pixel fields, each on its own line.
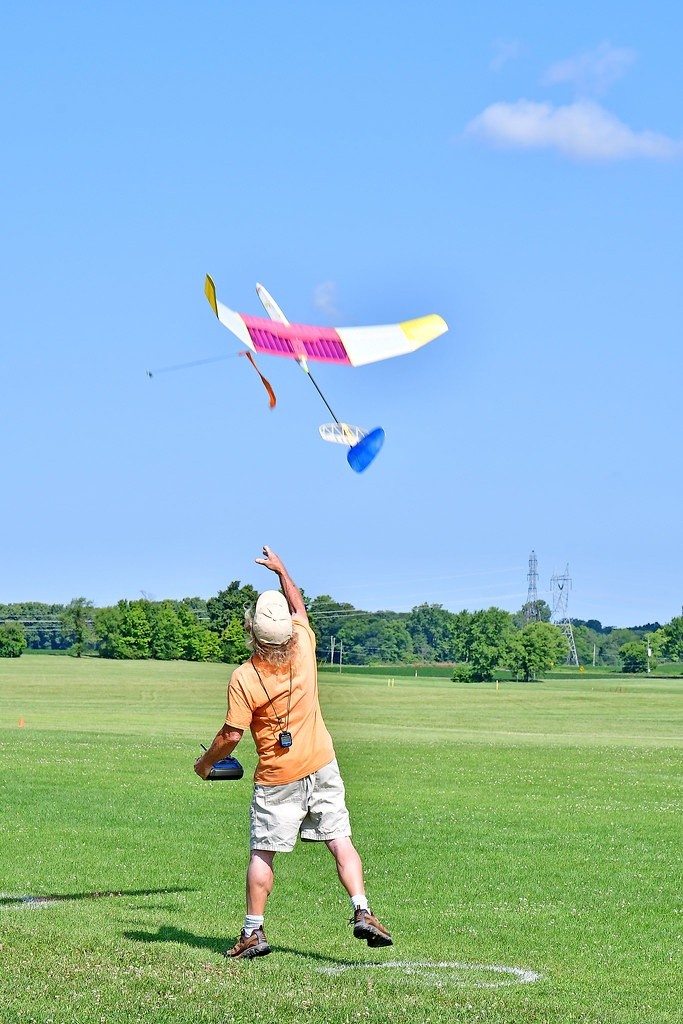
left=344, top=905, right=393, bottom=947
left=224, top=925, right=271, bottom=959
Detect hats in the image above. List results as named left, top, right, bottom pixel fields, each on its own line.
left=244, top=590, right=293, bottom=648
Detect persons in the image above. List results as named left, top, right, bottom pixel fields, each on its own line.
left=191, top=545, right=392, bottom=962
